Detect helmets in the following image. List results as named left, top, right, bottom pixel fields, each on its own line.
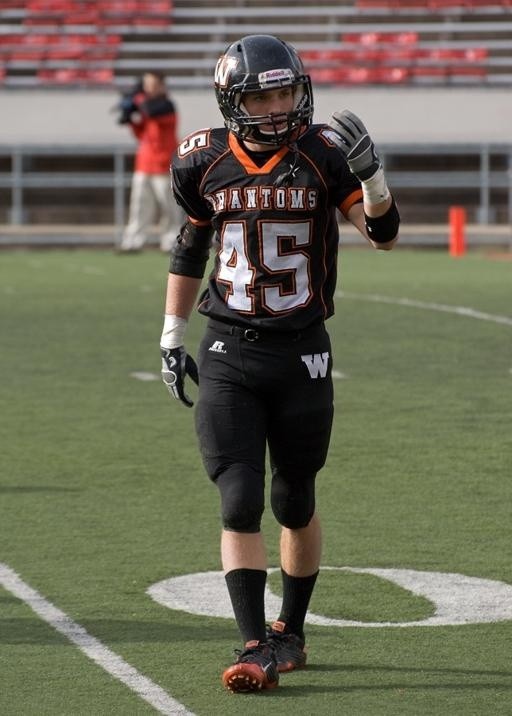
left=214, top=34, right=314, bottom=144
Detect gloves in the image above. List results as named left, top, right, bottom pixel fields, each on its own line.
left=320, top=109, right=385, bottom=182
left=160, top=344, right=199, bottom=408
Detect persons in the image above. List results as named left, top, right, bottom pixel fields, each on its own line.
left=158, top=29, right=403, bottom=697
left=115, top=66, right=192, bottom=259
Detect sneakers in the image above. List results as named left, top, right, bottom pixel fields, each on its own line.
left=262, top=621, right=306, bottom=671
left=221, top=638, right=279, bottom=693
